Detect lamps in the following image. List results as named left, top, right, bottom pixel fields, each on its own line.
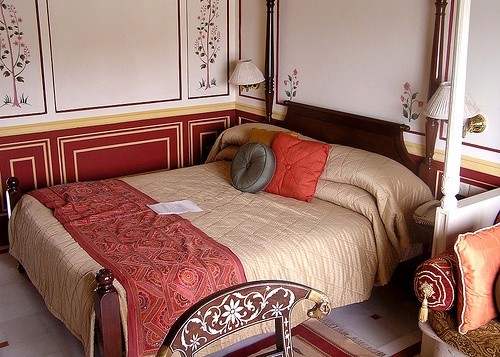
left=423, top=82, right=487, bottom=138
left=228, top=60, right=266, bottom=92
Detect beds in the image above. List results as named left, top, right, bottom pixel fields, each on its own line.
left=6, top=0, right=449, bottom=357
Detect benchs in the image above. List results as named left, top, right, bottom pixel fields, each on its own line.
left=418, top=188, right=500, bottom=357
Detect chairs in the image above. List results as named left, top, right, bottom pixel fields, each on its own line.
left=157, top=280, right=332, bottom=357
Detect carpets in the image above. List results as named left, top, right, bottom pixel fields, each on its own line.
left=224, top=317, right=389, bottom=357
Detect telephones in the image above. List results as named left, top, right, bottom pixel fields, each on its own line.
left=413, top=199, right=441, bottom=226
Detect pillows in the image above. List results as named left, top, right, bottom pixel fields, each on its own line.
left=454, top=222, right=500, bottom=335
left=248, top=126, right=298, bottom=147
left=230, top=141, right=276, bottom=193
left=263, top=132, right=331, bottom=203
left=414, top=248, right=457, bottom=323
